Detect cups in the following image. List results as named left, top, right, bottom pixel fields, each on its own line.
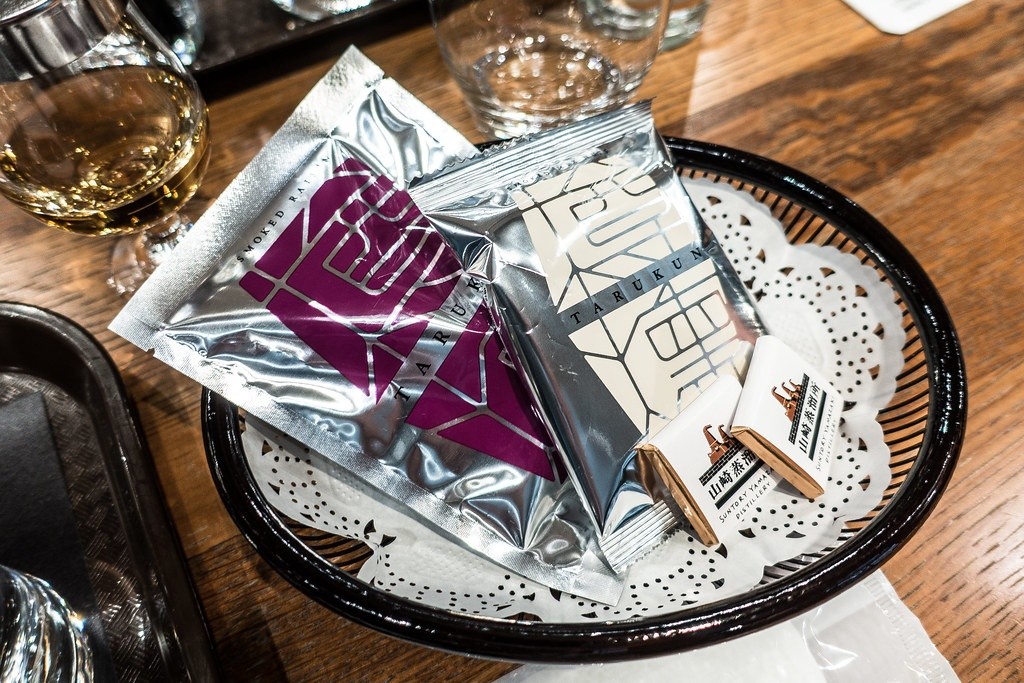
left=267, top=1, right=376, bottom=24
left=0, top=565, right=95, bottom=683
left=423, top=0, right=668, bottom=138
left=583, top=0, right=711, bottom=54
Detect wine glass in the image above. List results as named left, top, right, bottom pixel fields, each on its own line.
left=0, top=30, right=211, bottom=297
